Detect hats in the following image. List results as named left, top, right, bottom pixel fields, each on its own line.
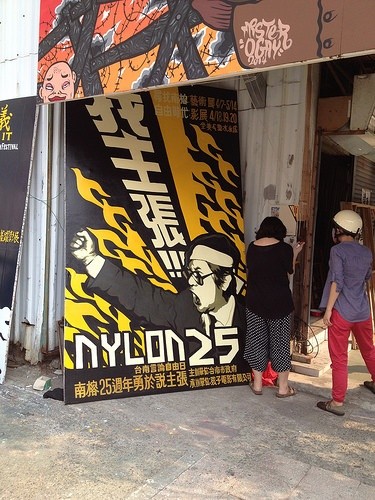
left=333, top=209, right=363, bottom=234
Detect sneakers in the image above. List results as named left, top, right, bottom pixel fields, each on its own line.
left=316, top=399, right=346, bottom=416
left=363, top=381, right=375, bottom=395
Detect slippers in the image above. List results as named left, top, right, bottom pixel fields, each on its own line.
left=277, top=385, right=298, bottom=398
left=249, top=381, right=263, bottom=395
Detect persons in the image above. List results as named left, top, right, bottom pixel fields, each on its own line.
left=317, top=210, right=375, bottom=415
left=244, top=215, right=305, bottom=398
